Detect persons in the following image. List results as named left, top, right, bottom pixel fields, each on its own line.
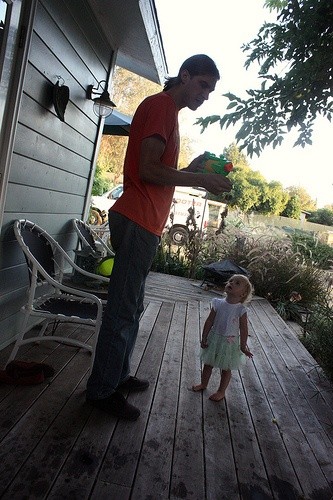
left=192, top=274, right=254, bottom=402
left=83, top=54, right=234, bottom=422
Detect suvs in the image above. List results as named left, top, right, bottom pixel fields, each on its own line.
left=90, top=181, right=210, bottom=245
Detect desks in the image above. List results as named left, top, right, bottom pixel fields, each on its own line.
left=43, top=273, right=110, bottom=347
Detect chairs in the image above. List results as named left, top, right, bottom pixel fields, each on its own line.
left=2, top=217, right=116, bottom=372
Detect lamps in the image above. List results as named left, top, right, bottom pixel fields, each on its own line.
left=86, top=80, right=117, bottom=119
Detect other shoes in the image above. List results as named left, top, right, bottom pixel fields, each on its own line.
left=118, top=376, right=150, bottom=391
left=88, top=393, right=141, bottom=419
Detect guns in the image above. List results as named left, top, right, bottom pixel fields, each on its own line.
left=196, top=151, right=233, bottom=177
